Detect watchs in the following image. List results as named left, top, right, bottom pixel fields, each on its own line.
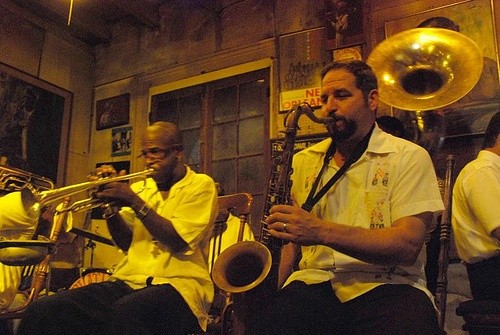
left=136, top=202, right=152, bottom=220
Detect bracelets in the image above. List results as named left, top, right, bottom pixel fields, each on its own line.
left=102, top=210, right=118, bottom=219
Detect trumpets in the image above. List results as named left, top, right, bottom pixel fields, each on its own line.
left=21, top=167, right=155, bottom=213
left=0, top=165, right=54, bottom=193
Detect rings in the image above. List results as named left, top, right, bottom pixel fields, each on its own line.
left=282, top=223, right=287, bottom=232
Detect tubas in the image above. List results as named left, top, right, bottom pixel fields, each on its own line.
left=365, top=27, right=484, bottom=137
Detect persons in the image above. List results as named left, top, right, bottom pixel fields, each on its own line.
left=244, top=59, right=447, bottom=335
left=13, top=122, right=219, bottom=335
left=451, top=112, right=500, bottom=335
left=0, top=151, right=40, bottom=313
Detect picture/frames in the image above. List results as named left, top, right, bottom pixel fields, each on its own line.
left=0, top=62, right=73, bottom=190
left=382, top=0, right=500, bottom=117
left=95, top=93, right=132, bottom=130
left=276, top=25, right=327, bottom=113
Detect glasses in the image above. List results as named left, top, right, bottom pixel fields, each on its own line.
left=136, top=145, right=183, bottom=162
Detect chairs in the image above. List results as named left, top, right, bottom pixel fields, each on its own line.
left=424, top=154, right=456, bottom=335
left=207, top=190, right=254, bottom=335
left=0, top=185, right=71, bottom=335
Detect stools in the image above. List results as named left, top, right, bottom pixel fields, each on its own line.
left=456, top=298, right=500, bottom=335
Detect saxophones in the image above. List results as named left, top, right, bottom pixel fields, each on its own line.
left=211, top=101, right=336, bottom=335
